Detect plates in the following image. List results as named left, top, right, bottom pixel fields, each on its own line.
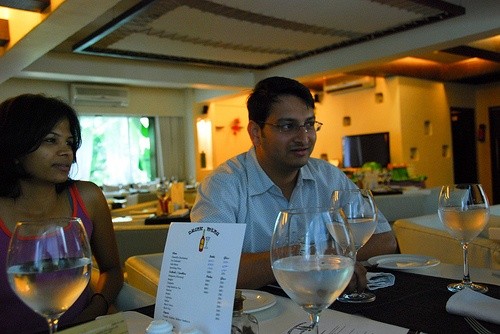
left=237, top=288, right=277, bottom=313
left=367, top=254, right=441, bottom=269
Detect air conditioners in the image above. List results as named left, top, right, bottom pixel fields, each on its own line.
left=324, top=75, right=375, bottom=96
left=70, top=82, right=131, bottom=109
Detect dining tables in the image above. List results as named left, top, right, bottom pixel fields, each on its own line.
left=57, top=252, right=500, bottom=334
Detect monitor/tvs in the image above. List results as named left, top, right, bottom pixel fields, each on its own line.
left=343, top=132, right=390, bottom=168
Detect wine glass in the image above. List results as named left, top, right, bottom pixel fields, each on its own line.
left=6, top=217, right=92, bottom=334
left=270, top=207, right=355, bottom=334
left=439, top=184, right=489, bottom=294
left=326, top=189, right=376, bottom=302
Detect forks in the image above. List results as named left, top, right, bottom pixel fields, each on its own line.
left=464, top=315, right=492, bottom=334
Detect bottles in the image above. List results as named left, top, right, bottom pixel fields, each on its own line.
left=230, top=290, right=259, bottom=334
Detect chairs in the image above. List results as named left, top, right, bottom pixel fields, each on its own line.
left=122, top=248, right=164, bottom=293
left=106, top=190, right=198, bottom=251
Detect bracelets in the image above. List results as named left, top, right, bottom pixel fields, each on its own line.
left=89, top=293, right=109, bottom=311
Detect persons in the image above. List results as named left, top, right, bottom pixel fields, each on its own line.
left=190, top=76, right=397, bottom=290
left=0, top=93, right=124, bottom=334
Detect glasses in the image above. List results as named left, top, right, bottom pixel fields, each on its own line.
left=253, top=119, right=323, bottom=133
